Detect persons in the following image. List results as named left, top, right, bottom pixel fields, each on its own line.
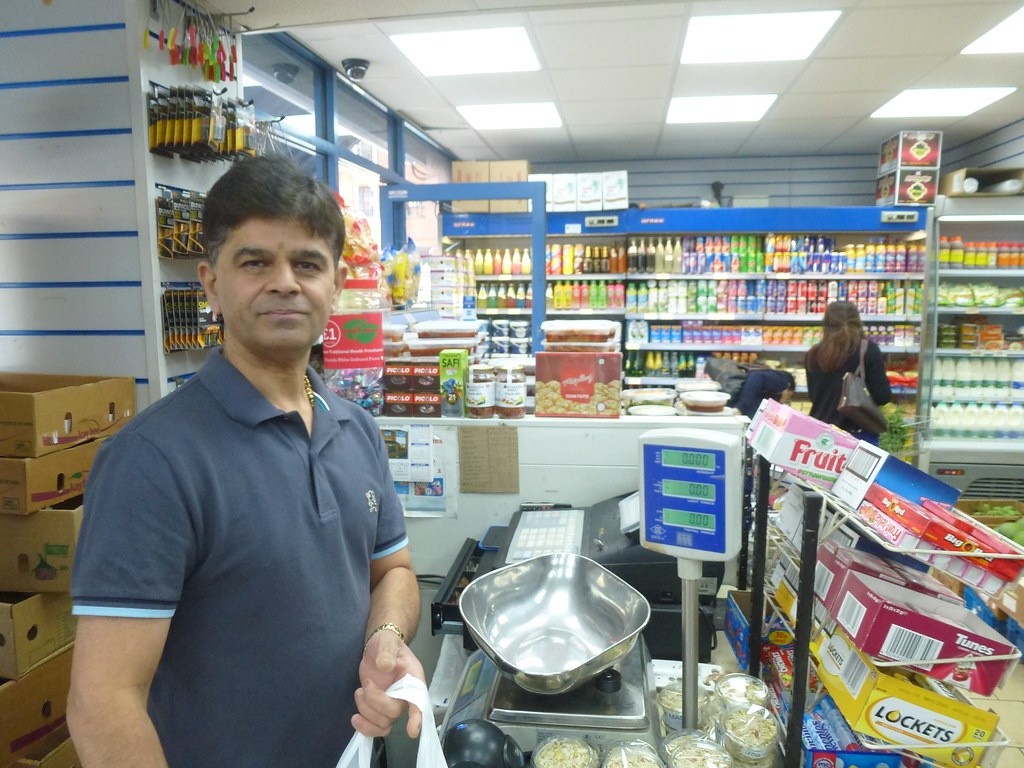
left=733, top=370, right=796, bottom=419
left=66, top=153, right=421, bottom=768
left=804, top=300, right=892, bottom=446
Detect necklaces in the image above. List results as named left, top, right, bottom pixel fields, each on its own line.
left=304, top=375, right=314, bottom=410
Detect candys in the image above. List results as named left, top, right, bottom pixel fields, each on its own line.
left=323, top=366, right=384, bottom=417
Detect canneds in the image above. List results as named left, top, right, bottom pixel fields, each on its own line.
left=681, top=234, right=848, bottom=273
left=646, top=279, right=888, bottom=314
left=468, top=364, right=527, bottom=419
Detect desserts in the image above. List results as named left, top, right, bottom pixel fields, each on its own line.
left=529, top=674, right=783, bottom=768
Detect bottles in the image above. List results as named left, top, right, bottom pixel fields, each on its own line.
left=474, top=280, right=668, bottom=314
left=495, top=366, right=528, bottom=419
left=586, top=233, right=682, bottom=274
left=322, top=278, right=384, bottom=415
left=922, top=357, right=1024, bottom=439
left=455, top=244, right=532, bottom=275
left=624, top=352, right=758, bottom=379
left=466, top=365, right=495, bottom=418
left=844, top=233, right=1024, bottom=271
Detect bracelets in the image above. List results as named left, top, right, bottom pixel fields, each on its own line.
left=363, top=622, right=405, bottom=655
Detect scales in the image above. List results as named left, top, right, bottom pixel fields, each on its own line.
left=438, top=427, right=747, bottom=768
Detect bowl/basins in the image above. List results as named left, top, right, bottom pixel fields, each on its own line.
left=533, top=673, right=783, bottom=768
left=383, top=320, right=490, bottom=364
left=628, top=405, right=682, bottom=415
left=675, top=380, right=738, bottom=417
left=492, top=319, right=533, bottom=356
left=624, top=387, right=678, bottom=415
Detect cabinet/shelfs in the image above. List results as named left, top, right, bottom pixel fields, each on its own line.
left=439, top=211, right=1024, bottom=460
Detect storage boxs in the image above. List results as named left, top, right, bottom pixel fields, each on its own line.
left=874, top=130, right=1024, bottom=206
left=726, top=396, right=1024, bottom=768
left=0, top=367, right=137, bottom=768
left=450, top=159, right=628, bottom=213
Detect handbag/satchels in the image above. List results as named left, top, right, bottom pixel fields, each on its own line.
left=837, top=372, right=888, bottom=436
left=705, top=358, right=747, bottom=400
